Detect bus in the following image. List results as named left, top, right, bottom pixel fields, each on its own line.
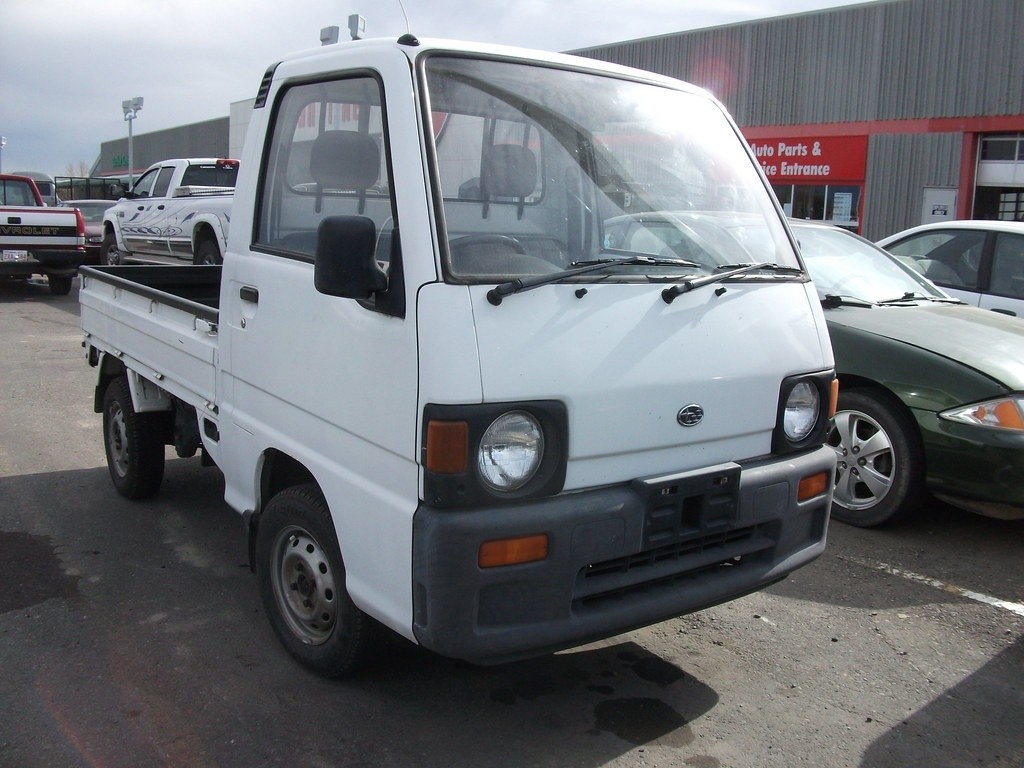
left=54, top=174, right=142, bottom=201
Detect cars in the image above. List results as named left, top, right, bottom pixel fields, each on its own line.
left=293, top=183, right=381, bottom=195
left=602, top=210, right=1024, bottom=529
left=55, top=200, right=118, bottom=265
left=802, top=220, right=1024, bottom=320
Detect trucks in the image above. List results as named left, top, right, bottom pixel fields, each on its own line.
left=79, top=35, right=838, bottom=679
left=0, top=171, right=59, bottom=207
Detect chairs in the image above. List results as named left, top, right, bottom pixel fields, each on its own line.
left=893, top=254, right=977, bottom=285
left=448, top=144, right=568, bottom=268
left=281, top=130, right=389, bottom=261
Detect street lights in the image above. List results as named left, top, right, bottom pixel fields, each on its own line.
left=122, top=97, right=143, bottom=192
left=0, top=136, right=7, bottom=174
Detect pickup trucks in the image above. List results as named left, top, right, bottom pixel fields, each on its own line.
left=101, top=157, right=242, bottom=267
left=0, top=174, right=86, bottom=295
